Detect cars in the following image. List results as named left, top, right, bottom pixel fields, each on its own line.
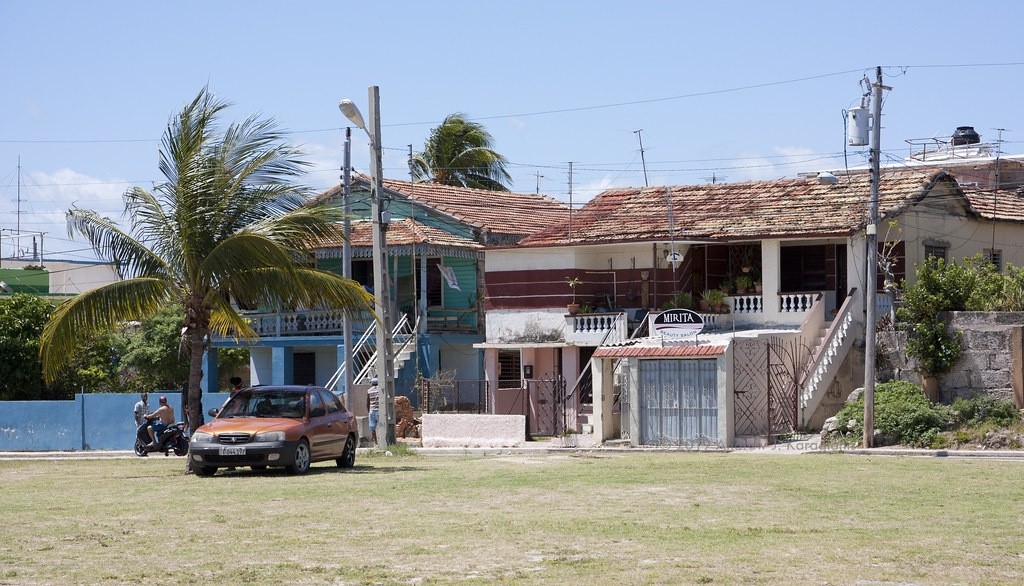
left=184, top=383, right=362, bottom=475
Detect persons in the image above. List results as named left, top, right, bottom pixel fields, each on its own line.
left=144, top=396, right=174, bottom=456
left=226, top=377, right=247, bottom=414
left=182, top=369, right=204, bottom=425
left=134, top=393, right=150, bottom=427
left=366, top=378, right=379, bottom=440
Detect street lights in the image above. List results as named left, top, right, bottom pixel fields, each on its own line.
left=337, top=84, right=404, bottom=447
left=816, top=172, right=876, bottom=449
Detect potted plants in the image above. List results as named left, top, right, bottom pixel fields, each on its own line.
left=698, top=276, right=762, bottom=313
left=740, top=256, right=754, bottom=273
left=565, top=276, right=583, bottom=313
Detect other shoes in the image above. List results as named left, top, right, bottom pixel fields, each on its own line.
left=147, top=442, right=157, bottom=447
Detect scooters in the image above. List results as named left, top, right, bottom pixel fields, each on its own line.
left=132, top=409, right=191, bottom=457
